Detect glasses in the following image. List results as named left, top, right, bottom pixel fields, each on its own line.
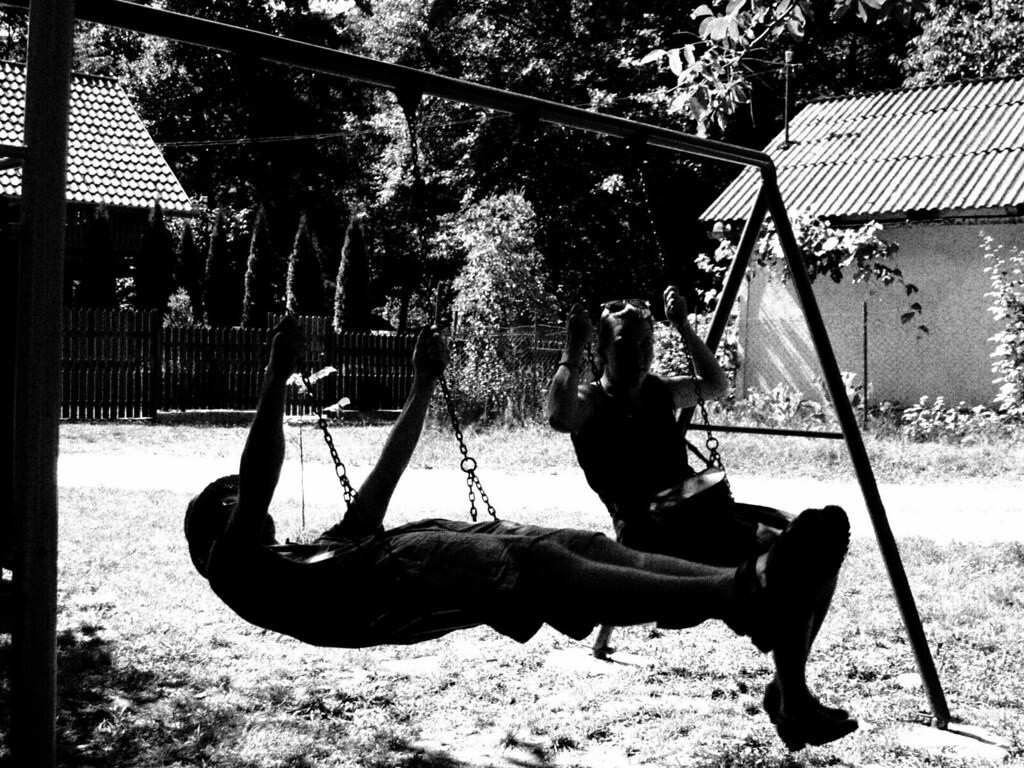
left=607, top=299, right=649, bottom=312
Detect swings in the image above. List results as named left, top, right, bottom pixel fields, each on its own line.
left=528, top=114, right=740, bottom=541
left=233, top=55, right=503, bottom=638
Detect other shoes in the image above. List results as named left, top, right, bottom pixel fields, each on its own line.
left=729, top=507, right=849, bottom=653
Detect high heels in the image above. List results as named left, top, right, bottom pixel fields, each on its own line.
left=776, top=703, right=858, bottom=752
left=763, top=683, right=848, bottom=725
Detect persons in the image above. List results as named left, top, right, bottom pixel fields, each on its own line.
left=185, top=310, right=793, bottom=651
left=546, top=287, right=859, bottom=750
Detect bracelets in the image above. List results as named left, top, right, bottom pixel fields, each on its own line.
left=555, top=360, right=585, bottom=374
